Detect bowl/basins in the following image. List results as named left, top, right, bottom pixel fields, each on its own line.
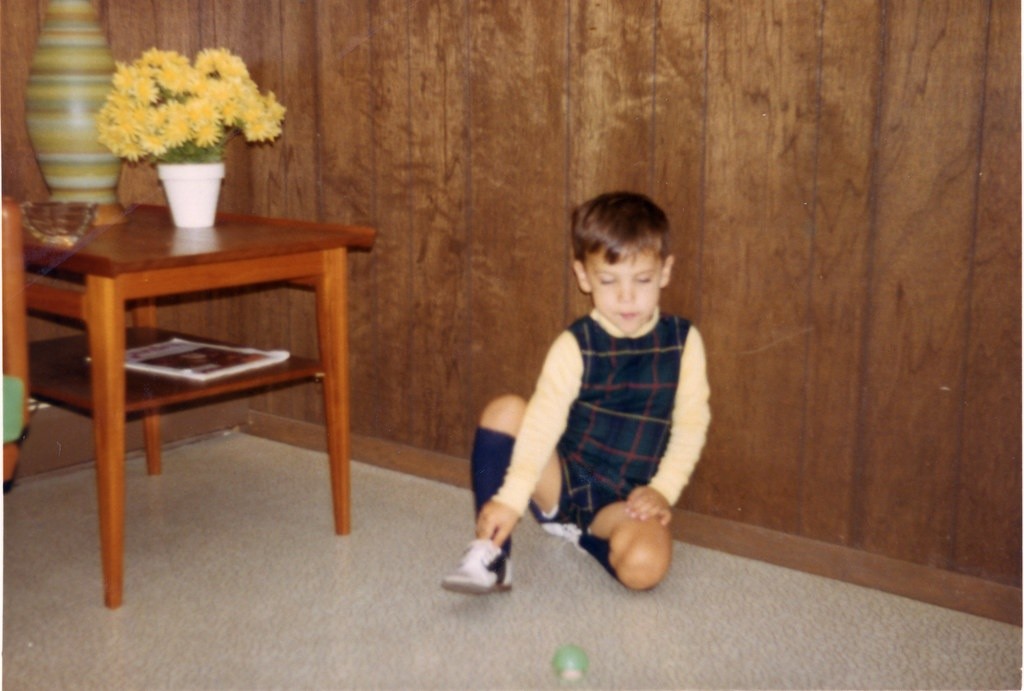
left=20, top=201, right=94, bottom=242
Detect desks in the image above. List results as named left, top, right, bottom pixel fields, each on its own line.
left=23, top=202, right=378, bottom=609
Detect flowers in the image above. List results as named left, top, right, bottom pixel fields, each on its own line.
left=97, top=44, right=289, bottom=164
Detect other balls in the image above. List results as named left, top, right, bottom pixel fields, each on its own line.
left=553, top=644, right=587, bottom=679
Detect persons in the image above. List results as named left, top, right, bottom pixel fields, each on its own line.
left=441, top=191, right=711, bottom=595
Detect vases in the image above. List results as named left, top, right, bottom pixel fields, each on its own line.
left=157, top=163, right=226, bottom=228
left=26, top=1, right=128, bottom=227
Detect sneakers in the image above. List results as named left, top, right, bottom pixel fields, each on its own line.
left=542, top=522, right=589, bottom=554
left=441, top=538, right=512, bottom=594
